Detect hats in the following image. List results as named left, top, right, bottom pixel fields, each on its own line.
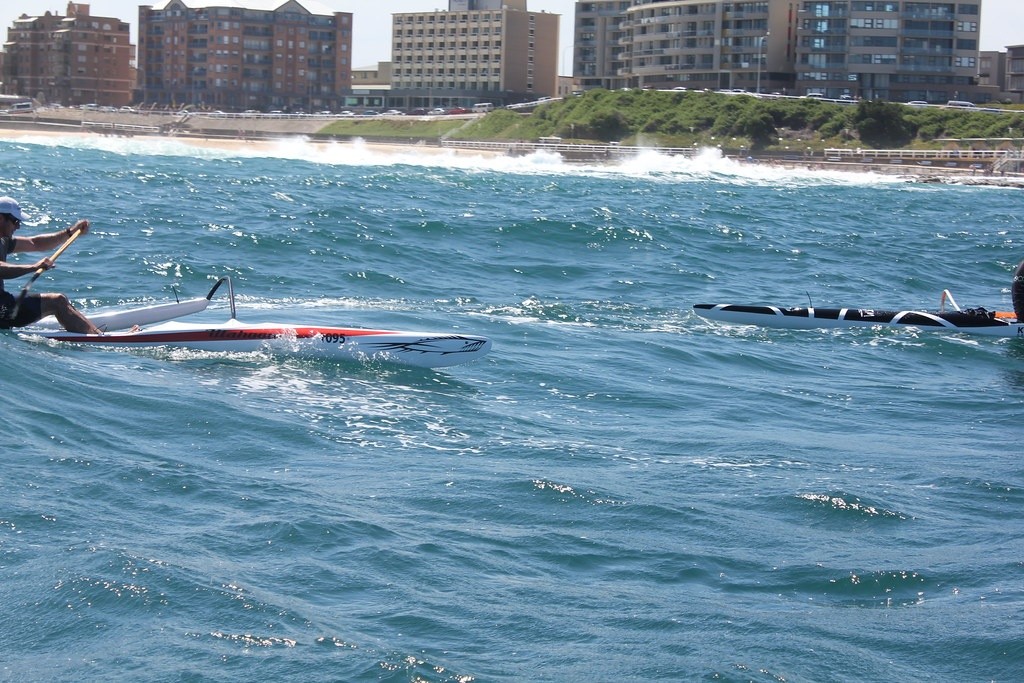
left=0, top=195, right=32, bottom=221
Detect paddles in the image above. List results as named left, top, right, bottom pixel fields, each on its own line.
left=6, top=226, right=84, bottom=324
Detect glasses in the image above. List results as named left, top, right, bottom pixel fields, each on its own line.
left=5, top=215, right=21, bottom=225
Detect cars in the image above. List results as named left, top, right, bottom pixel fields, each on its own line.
left=0, top=87, right=1006, bottom=116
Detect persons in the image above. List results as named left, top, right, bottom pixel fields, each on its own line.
left=1012, top=260, right=1024, bottom=324
left=0, top=195, right=139, bottom=335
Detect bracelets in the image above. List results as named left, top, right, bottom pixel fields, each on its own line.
left=66, top=228, right=72, bottom=237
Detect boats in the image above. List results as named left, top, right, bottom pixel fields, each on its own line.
left=29, top=271, right=236, bottom=330
left=11, top=317, right=493, bottom=369
left=694, top=302, right=1023, bottom=338
